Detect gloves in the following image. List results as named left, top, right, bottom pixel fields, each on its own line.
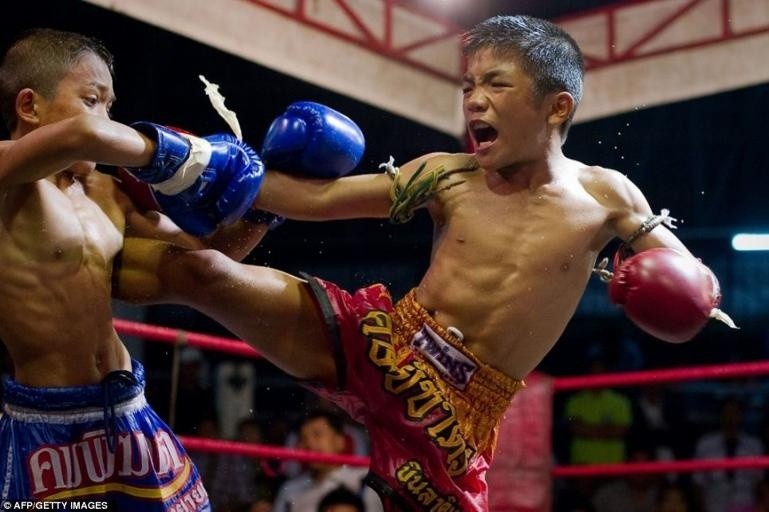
left=126, top=122, right=265, bottom=238
left=609, top=247, right=721, bottom=343
left=241, top=101, right=365, bottom=228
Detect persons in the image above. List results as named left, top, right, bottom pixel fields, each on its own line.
left=112, top=15, right=722, bottom=512
left=562, top=357, right=767, bottom=512
left=0, top=28, right=367, bottom=512
left=178, top=349, right=385, bottom=512
